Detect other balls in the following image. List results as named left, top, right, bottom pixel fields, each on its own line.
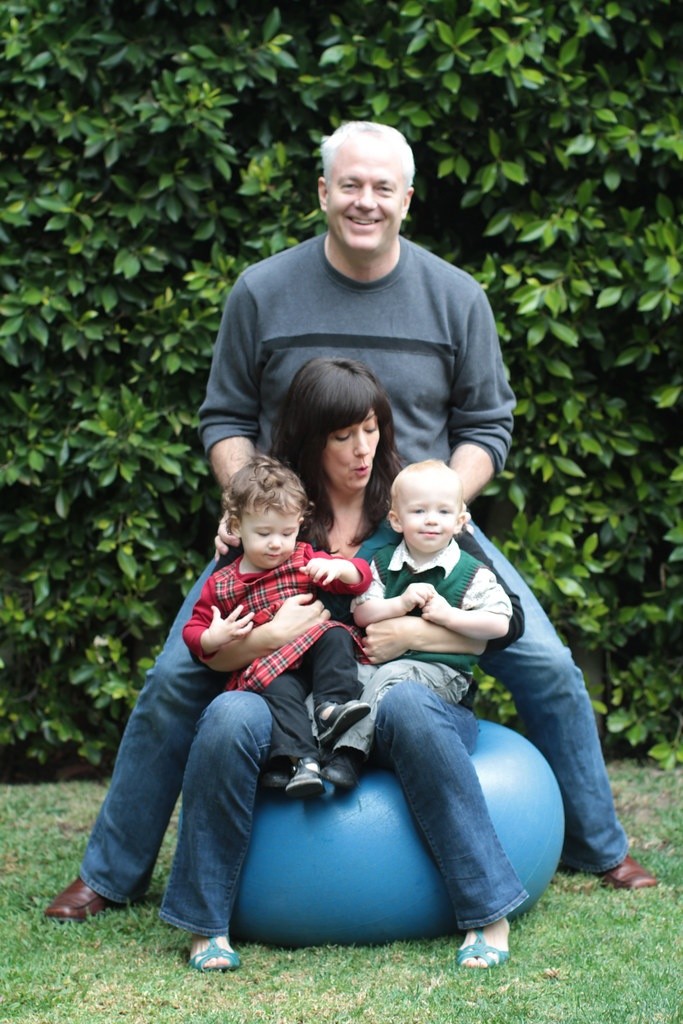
left=176, top=715, right=568, bottom=950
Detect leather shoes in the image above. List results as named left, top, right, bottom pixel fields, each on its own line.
left=321, top=750, right=366, bottom=788
left=263, top=759, right=291, bottom=787
left=46, top=878, right=110, bottom=920
left=602, top=855, right=656, bottom=891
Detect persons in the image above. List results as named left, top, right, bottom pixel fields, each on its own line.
left=182, top=459, right=371, bottom=796
left=261, top=459, right=513, bottom=789
left=159, top=356, right=525, bottom=969
left=46, top=121, right=657, bottom=921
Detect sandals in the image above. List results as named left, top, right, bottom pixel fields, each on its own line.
left=457, top=925, right=510, bottom=970
left=188, top=929, right=240, bottom=972
left=315, top=701, right=372, bottom=748
left=285, top=757, right=325, bottom=800
